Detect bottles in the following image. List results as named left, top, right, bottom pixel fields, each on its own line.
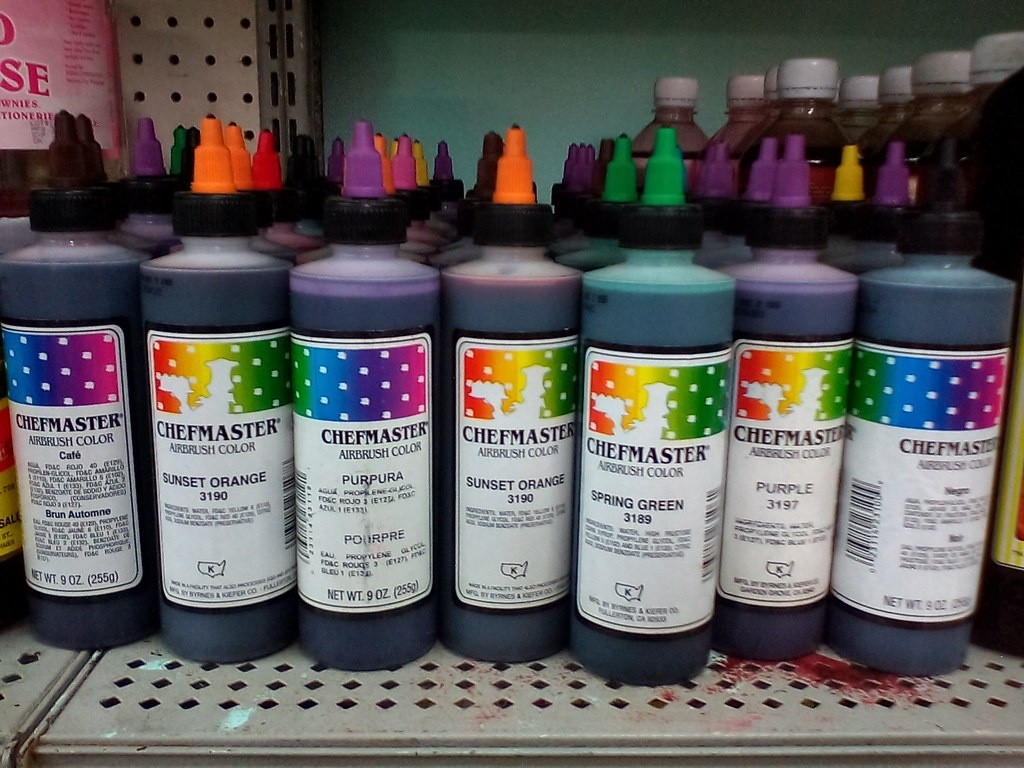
left=0, top=35, right=1024, bottom=684
